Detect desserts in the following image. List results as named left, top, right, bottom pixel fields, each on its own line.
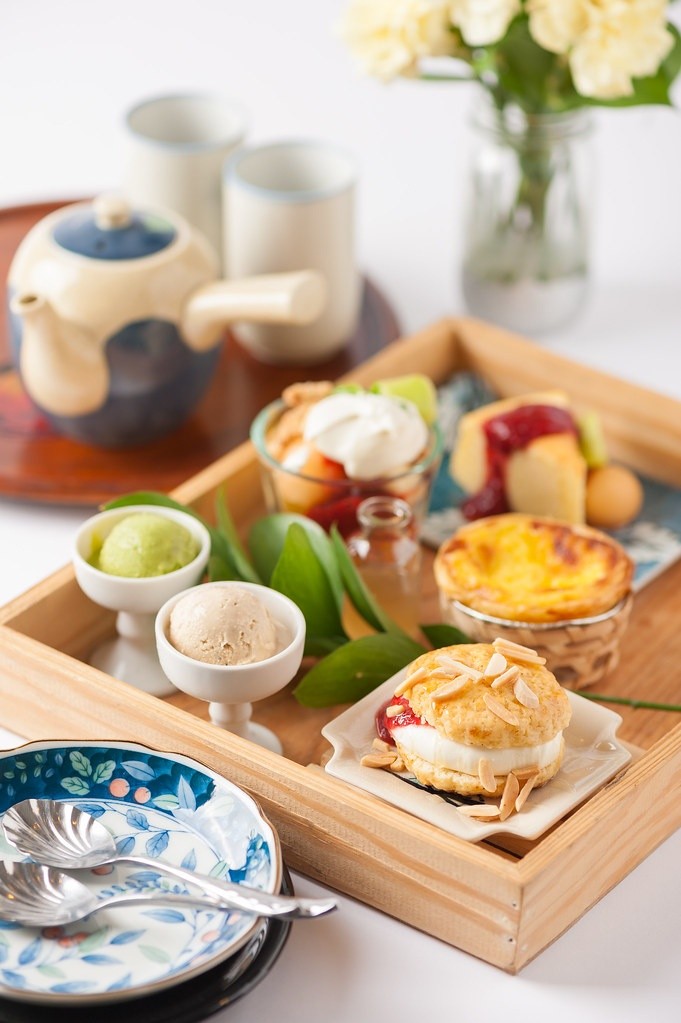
left=359, top=637, right=573, bottom=824
left=99, top=511, right=276, bottom=667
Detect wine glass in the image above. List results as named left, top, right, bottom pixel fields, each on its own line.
left=72, top=505, right=211, bottom=697
left=156, top=577, right=306, bottom=757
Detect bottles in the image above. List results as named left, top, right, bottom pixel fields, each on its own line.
left=343, top=496, right=424, bottom=611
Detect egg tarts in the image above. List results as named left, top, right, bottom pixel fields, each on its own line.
left=431, top=512, right=634, bottom=623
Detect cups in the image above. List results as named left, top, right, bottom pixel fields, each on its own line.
left=250, top=393, right=445, bottom=599
left=218, top=139, right=364, bottom=367
left=118, top=91, right=251, bottom=251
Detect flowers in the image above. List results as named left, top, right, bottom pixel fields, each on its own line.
left=345, top=0, right=680, bottom=245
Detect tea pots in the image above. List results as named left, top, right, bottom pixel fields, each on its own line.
left=3, top=188, right=326, bottom=450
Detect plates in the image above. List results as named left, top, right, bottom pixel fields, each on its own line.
left=0, top=865, right=297, bottom=1023
left=0, top=738, right=277, bottom=1005
left=419, top=369, right=681, bottom=597
left=314, top=650, right=629, bottom=845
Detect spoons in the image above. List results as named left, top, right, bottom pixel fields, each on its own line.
left=0, top=854, right=340, bottom=930
left=5, top=794, right=344, bottom=924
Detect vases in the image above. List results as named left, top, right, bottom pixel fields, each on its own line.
left=459, top=93, right=597, bottom=337
